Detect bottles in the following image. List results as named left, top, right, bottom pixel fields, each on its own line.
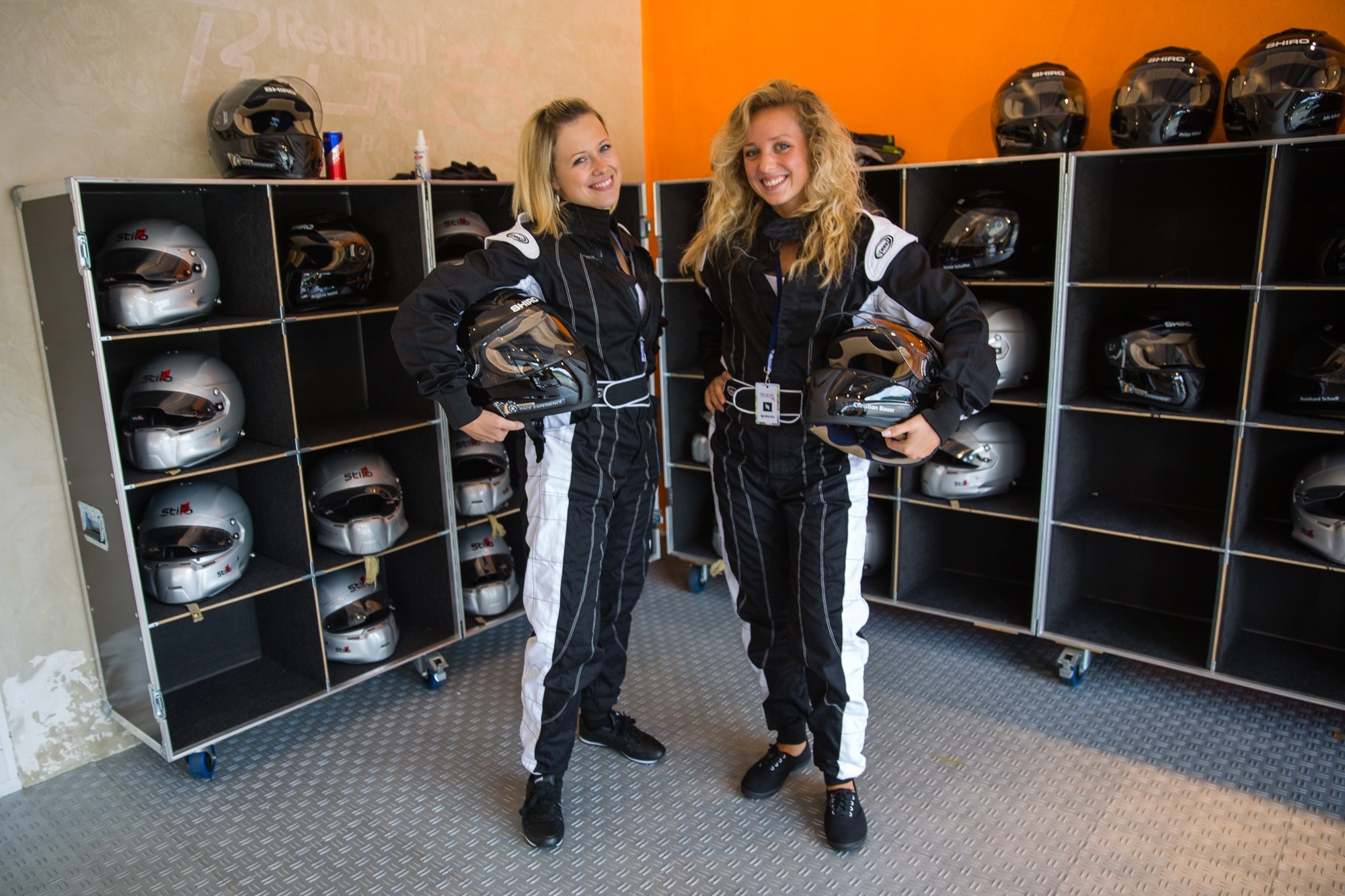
left=414, top=130, right=432, bottom=181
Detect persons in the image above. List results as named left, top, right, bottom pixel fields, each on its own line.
left=390, top=96, right=668, bottom=854
left=679, top=82, right=1001, bottom=856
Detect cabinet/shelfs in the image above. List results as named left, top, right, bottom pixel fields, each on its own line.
left=11, top=173, right=661, bottom=775
left=653, top=132, right=1344, bottom=711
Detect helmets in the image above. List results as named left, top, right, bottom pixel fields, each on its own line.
left=922, top=415, right=1024, bottom=500
left=1323, top=234, right=1345, bottom=281
left=1222, top=29, right=1345, bottom=141
left=850, top=133, right=905, bottom=167
left=979, top=301, right=1040, bottom=391
left=450, top=433, right=513, bottom=516
left=805, top=312, right=944, bottom=464
left=1289, top=451, right=1345, bottom=566
left=1289, top=322, right=1345, bottom=419
left=317, top=569, right=399, bottom=663
left=93, top=219, right=218, bottom=328
left=1110, top=46, right=1222, bottom=150
left=433, top=209, right=492, bottom=242
left=122, top=349, right=244, bottom=469
left=937, top=206, right=1038, bottom=281
left=713, top=524, right=722, bottom=556
left=307, top=452, right=408, bottom=557
left=283, top=223, right=374, bottom=313
left=457, top=289, right=600, bottom=422
left=208, top=76, right=324, bottom=180
left=135, top=482, right=252, bottom=604
left=1102, top=321, right=1208, bottom=413
left=861, top=511, right=887, bottom=577
left=991, top=62, right=1088, bottom=157
left=690, top=410, right=713, bottom=462
left=458, top=526, right=519, bottom=616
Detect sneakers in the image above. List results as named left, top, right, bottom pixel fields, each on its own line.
left=518, top=773, right=565, bottom=848
left=824, top=780, right=868, bottom=853
left=579, top=710, right=666, bottom=764
left=741, top=739, right=811, bottom=800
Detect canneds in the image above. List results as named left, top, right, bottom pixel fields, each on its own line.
left=323, top=131, right=347, bottom=180
